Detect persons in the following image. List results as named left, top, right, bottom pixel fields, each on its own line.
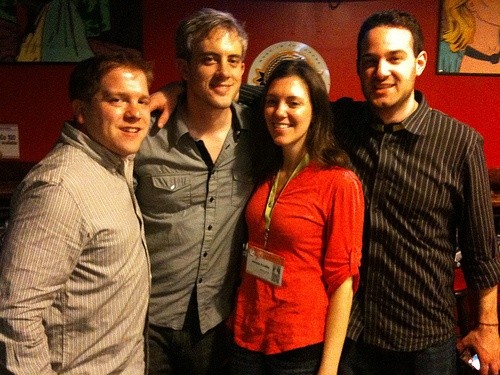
left=0, top=42, right=150, bottom=375
left=148, top=8, right=500, bottom=375
left=132, top=9, right=283, bottom=375
left=225, top=57, right=365, bottom=375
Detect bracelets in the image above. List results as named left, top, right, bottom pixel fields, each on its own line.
left=480, top=322, right=499, bottom=326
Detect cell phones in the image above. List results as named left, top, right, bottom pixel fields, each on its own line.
left=459, top=345, right=492, bottom=374
